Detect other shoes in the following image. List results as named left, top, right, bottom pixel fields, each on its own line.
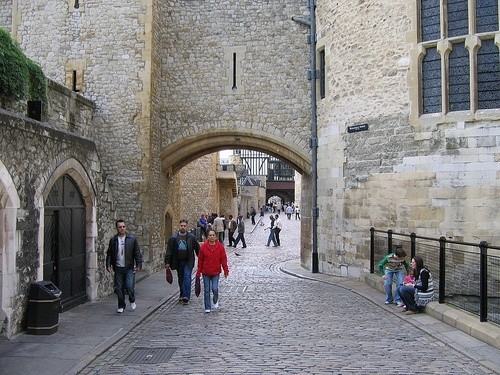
left=183, top=297, right=189, bottom=304
left=233, top=246, right=235, bottom=247
left=213, top=302, right=218, bottom=308
left=205, top=309, right=211, bottom=313
left=385, top=301, right=393, bottom=304
left=397, top=303, right=405, bottom=307
left=242, top=246, right=246, bottom=248
left=405, top=310, right=419, bottom=315
left=179, top=297, right=183, bottom=300
left=401, top=308, right=408, bottom=312
left=228, top=245, right=232, bottom=246
left=396, top=302, right=400, bottom=304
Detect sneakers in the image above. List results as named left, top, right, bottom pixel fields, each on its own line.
left=117, top=308, right=124, bottom=313
left=130, top=302, right=136, bottom=310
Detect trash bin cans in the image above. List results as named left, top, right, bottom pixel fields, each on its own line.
left=26, top=281, right=63, bottom=335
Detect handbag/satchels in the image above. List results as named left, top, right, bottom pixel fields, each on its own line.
left=165, top=268, right=173, bottom=284
left=195, top=278, right=200, bottom=296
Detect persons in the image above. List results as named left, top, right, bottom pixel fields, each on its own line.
left=259, top=207, right=265, bottom=226
left=197, top=212, right=226, bottom=244
left=227, top=215, right=237, bottom=246
left=270, top=214, right=282, bottom=246
left=397, top=268, right=415, bottom=308
left=165, top=219, right=200, bottom=305
left=295, top=206, right=301, bottom=220
left=397, top=256, right=434, bottom=315
left=233, top=216, right=247, bottom=248
left=378, top=248, right=411, bottom=305
left=106, top=220, right=143, bottom=313
left=250, top=206, right=256, bottom=225
left=196, top=229, right=229, bottom=313
left=266, top=202, right=295, bottom=220
left=264, top=214, right=278, bottom=247
